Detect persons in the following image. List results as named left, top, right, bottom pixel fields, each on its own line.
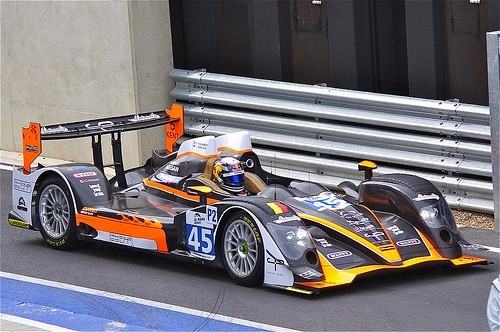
left=212, top=156, right=260, bottom=197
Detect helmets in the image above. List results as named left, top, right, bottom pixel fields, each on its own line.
left=212, top=157, right=245, bottom=193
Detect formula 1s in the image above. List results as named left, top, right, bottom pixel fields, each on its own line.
left=8, top=103, right=495, bottom=296
